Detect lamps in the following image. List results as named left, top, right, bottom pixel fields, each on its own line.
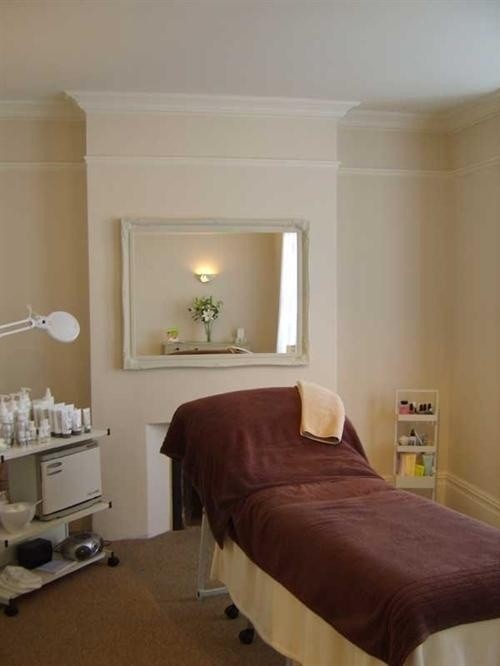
left=0, top=304, right=81, bottom=343
left=196, top=274, right=216, bottom=284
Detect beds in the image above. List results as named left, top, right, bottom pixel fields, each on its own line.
left=160, top=388, right=499, bottom=666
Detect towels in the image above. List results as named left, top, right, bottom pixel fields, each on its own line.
left=295, top=380, right=345, bottom=445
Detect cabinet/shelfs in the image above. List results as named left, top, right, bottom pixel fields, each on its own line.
left=394, top=388, right=439, bottom=489
left=0, top=428, right=120, bottom=617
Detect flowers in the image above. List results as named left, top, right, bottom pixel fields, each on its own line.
left=189, top=295, right=224, bottom=322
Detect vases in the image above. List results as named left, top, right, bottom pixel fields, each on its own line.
left=202, top=322, right=214, bottom=344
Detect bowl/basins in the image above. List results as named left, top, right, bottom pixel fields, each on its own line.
left=0, top=501, right=36, bottom=535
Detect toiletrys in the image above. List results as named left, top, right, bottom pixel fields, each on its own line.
left=0, top=384, right=92, bottom=445
left=400, top=399, right=436, bottom=415
left=398, top=428, right=434, bottom=446
left=397, top=454, right=435, bottom=477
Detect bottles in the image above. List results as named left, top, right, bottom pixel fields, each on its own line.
left=399, top=400, right=433, bottom=416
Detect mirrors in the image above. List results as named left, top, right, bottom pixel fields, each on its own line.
left=120, top=216, right=309, bottom=370
